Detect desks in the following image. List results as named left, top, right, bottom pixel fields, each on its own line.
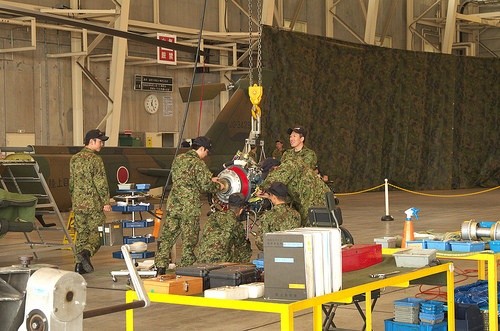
left=382, top=247, right=500, bottom=331
left=126, top=261, right=455, bottom=331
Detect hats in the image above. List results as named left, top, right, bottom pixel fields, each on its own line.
left=86, top=129, right=110, bottom=141
left=286, top=126, right=307, bottom=137
left=229, top=192, right=249, bottom=206
left=269, top=182, right=287, bottom=197
left=196, top=136, right=214, bottom=152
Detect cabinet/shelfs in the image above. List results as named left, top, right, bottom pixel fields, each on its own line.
left=110, top=190, right=157, bottom=282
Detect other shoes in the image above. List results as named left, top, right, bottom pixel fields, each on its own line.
left=75, top=263, right=86, bottom=275
left=76, top=252, right=94, bottom=273
left=157, top=267, right=166, bottom=274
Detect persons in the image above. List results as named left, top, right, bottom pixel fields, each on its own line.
left=155, top=125, right=331, bottom=278
left=68, top=130, right=112, bottom=273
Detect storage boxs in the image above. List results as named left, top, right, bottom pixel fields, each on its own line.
left=117, top=183, right=151, bottom=189
left=340, top=244, right=383, bottom=272
left=105, top=223, right=123, bottom=246
left=406, top=239, right=485, bottom=253
left=119, top=134, right=135, bottom=146
left=141, top=262, right=263, bottom=297
left=384, top=298, right=483, bottom=331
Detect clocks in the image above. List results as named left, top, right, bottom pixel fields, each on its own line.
left=144, top=94, right=159, bottom=114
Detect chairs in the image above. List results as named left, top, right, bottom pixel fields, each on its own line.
left=308, top=207, right=354, bottom=246
